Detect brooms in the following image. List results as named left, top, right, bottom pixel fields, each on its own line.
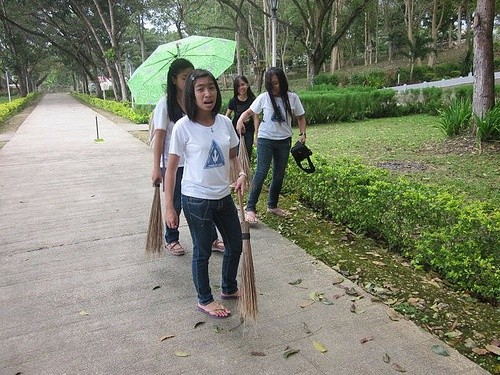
left=144, top=181, right=164, bottom=259
left=230, top=135, right=252, bottom=184
left=232, top=161, right=259, bottom=320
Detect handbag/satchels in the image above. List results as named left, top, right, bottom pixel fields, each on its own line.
left=291, top=141, right=315, bottom=174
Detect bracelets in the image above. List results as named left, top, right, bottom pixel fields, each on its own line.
left=239, top=172, right=247, bottom=178
left=299, top=132, right=306, bottom=138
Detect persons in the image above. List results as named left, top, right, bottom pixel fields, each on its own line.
left=236, top=66, right=306, bottom=223
left=165, top=69, right=247, bottom=317
left=152, top=58, right=225, bottom=256
left=225, top=74, right=259, bottom=164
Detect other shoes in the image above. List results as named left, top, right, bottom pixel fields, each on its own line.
left=212, top=239, right=225, bottom=252
left=165, top=241, right=184, bottom=255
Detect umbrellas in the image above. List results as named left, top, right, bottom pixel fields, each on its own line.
left=127, top=35, right=237, bottom=105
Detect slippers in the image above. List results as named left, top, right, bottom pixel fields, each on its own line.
left=245, top=210, right=258, bottom=223
left=197, top=305, right=231, bottom=318
left=267, top=207, right=286, bottom=216
left=221, top=293, right=238, bottom=300
